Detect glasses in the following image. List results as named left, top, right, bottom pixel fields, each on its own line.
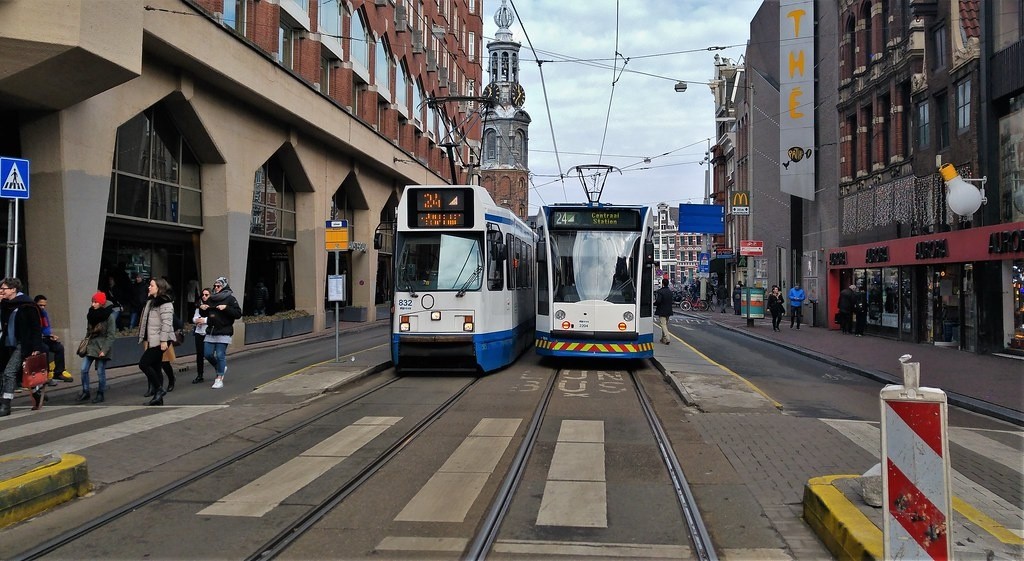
left=214, top=285, right=220, bottom=287
left=202, top=294, right=209, bottom=296
left=1, top=288, right=13, bottom=292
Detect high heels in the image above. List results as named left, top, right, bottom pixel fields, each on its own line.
left=93, top=391, right=104, bottom=402
left=76, top=392, right=91, bottom=402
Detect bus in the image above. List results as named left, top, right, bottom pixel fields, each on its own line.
left=373, top=92, right=539, bottom=374
left=534, top=163, right=660, bottom=360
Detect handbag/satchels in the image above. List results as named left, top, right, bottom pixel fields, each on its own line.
left=78, top=338, right=88, bottom=358
left=834, top=313, right=841, bottom=324
left=173, top=333, right=183, bottom=346
left=781, top=305, right=785, bottom=315
left=22, top=352, right=48, bottom=388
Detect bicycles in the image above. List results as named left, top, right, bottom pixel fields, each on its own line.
left=674, top=286, right=723, bottom=312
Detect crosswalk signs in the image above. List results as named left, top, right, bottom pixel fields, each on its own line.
left=0, top=158, right=31, bottom=200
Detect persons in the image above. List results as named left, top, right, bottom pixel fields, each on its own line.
left=33, top=295, right=67, bottom=380
left=103, top=275, right=124, bottom=320
left=138, top=277, right=184, bottom=405
left=787, top=283, right=805, bottom=331
left=653, top=278, right=673, bottom=345
left=716, top=284, right=728, bottom=313
left=0, top=277, right=43, bottom=416
left=733, top=281, right=744, bottom=315
left=200, top=277, right=241, bottom=389
left=75, top=292, right=115, bottom=402
left=193, top=288, right=211, bottom=384
left=706, top=281, right=714, bottom=301
left=664, top=272, right=668, bottom=279
left=767, top=285, right=785, bottom=332
left=129, top=273, right=149, bottom=330
left=837, top=284, right=869, bottom=337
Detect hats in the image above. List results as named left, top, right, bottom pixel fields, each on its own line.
left=93, top=291, right=106, bottom=305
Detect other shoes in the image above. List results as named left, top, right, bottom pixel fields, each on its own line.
left=54, top=373, right=65, bottom=380
left=221, top=366, right=228, bottom=379
left=33, top=392, right=40, bottom=407
left=150, top=388, right=167, bottom=404
left=168, top=379, right=175, bottom=391
left=790, top=323, right=793, bottom=328
left=144, top=398, right=163, bottom=406
left=666, top=342, right=670, bottom=344
left=193, top=376, right=204, bottom=384
left=144, top=387, right=153, bottom=396
left=212, top=379, right=223, bottom=389
left=0, top=405, right=10, bottom=416
left=774, top=325, right=779, bottom=332
left=659, top=338, right=664, bottom=342
left=796, top=326, right=800, bottom=330
